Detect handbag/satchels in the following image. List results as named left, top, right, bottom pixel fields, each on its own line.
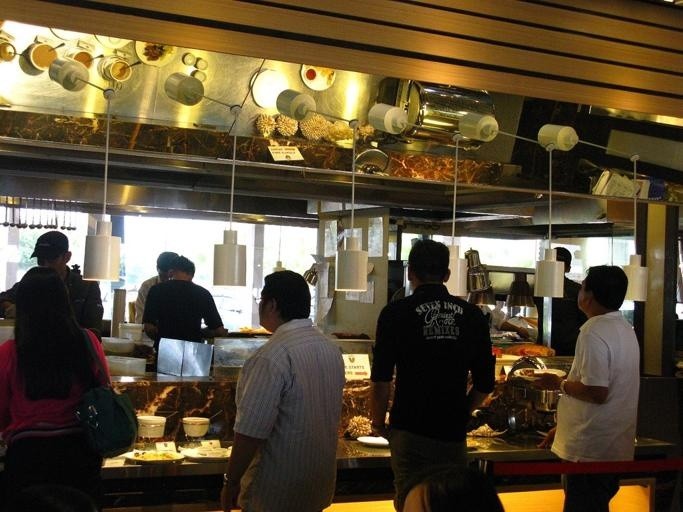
left=74, top=328, right=137, bottom=460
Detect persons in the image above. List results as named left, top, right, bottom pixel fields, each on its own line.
left=143, top=255, right=225, bottom=372
left=0, top=266, right=112, bottom=511
left=370, top=239, right=495, bottom=490
left=533, top=247, right=588, bottom=356
left=0, top=231, right=104, bottom=344
left=220, top=271, right=345, bottom=512
left=135, top=252, right=179, bottom=324
left=393, top=465, right=504, bottom=512
left=528, top=265, right=640, bottom=511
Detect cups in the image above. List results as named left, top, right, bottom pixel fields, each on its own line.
left=0, top=41, right=132, bottom=82
left=182, top=53, right=208, bottom=83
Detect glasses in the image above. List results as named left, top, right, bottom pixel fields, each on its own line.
left=254, top=296, right=262, bottom=304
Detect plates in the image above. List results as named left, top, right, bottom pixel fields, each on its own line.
left=300, top=63, right=337, bottom=91
left=181, top=446, right=231, bottom=462
left=355, top=435, right=390, bottom=448
left=124, top=450, right=184, bottom=465
left=466, top=440, right=481, bottom=449
left=134, top=40, right=178, bottom=66
left=513, top=367, right=567, bottom=382
left=465, top=428, right=507, bottom=437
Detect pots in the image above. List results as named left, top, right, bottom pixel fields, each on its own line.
left=526, top=388, right=558, bottom=413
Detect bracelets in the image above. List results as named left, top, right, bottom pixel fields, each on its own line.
left=559, top=378, right=567, bottom=395
left=222, top=474, right=239, bottom=487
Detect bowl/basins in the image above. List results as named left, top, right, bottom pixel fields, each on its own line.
left=136, top=414, right=167, bottom=440
left=100, top=337, right=135, bottom=354
left=182, top=417, right=211, bottom=437
left=103, top=356, right=146, bottom=376
left=117, top=322, right=142, bottom=341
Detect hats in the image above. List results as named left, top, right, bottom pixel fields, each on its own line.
left=30, top=230, right=70, bottom=261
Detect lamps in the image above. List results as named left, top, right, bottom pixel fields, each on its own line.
left=463, top=248, right=493, bottom=293
left=458, top=113, right=566, bottom=298
left=163, top=73, right=246, bottom=287
left=353, top=132, right=389, bottom=175
left=505, top=273, right=536, bottom=307
left=537, top=121, right=649, bottom=301
left=367, top=103, right=469, bottom=296
left=276, top=88, right=368, bottom=292
left=49, top=57, right=122, bottom=283
